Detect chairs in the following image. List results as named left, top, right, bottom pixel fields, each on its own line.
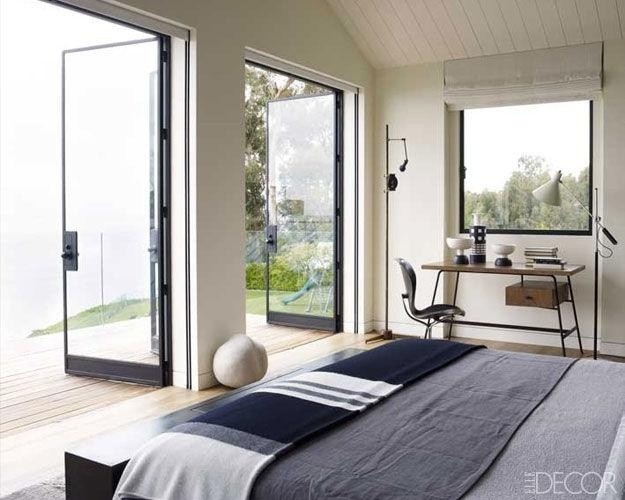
left=393, top=257, right=465, bottom=339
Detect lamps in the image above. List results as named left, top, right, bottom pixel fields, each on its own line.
left=532, top=170, right=617, bottom=359
left=365, top=124, right=420, bottom=343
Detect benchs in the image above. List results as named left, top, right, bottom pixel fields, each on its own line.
left=65, top=348, right=369, bottom=500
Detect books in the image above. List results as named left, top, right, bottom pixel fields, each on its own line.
left=524, top=246, right=567, bottom=270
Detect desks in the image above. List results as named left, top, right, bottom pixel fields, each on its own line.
left=422, top=261, right=585, bottom=358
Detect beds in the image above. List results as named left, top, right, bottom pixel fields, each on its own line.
left=113, top=336, right=625, bottom=500
